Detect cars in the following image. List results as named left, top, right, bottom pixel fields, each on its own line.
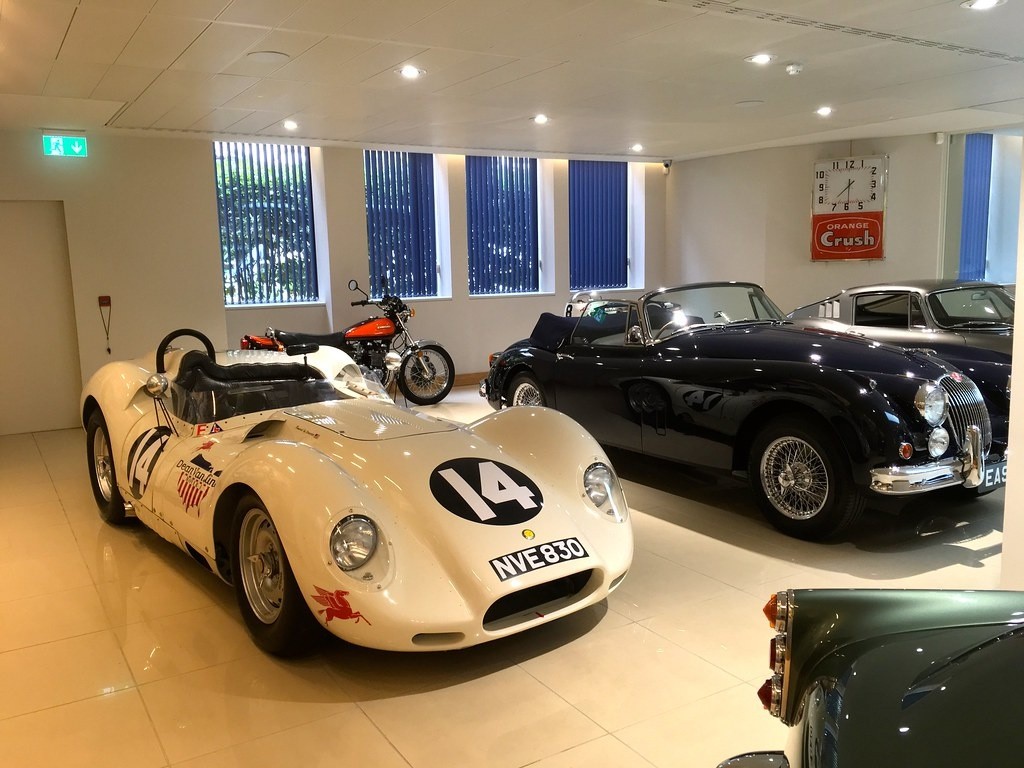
left=782, top=281, right=1016, bottom=356
left=709, top=586, right=1024, bottom=768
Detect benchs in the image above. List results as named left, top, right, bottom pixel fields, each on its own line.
left=174, top=353, right=335, bottom=419
left=531, top=303, right=695, bottom=344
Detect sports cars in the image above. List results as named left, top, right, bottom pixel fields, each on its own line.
left=74, top=327, right=638, bottom=663
left=476, top=278, right=1013, bottom=544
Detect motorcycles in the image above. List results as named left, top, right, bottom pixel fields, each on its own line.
left=239, top=279, right=457, bottom=406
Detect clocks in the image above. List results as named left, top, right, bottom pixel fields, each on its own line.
left=809, top=153, right=888, bottom=215
